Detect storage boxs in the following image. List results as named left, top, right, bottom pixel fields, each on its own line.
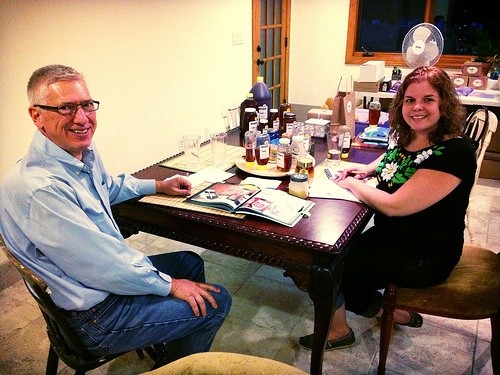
left=450, top=60, right=488, bottom=90
left=354, top=59, right=386, bottom=93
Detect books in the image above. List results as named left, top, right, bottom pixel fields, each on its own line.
left=349, top=131, right=390, bottom=150
left=350, top=124, right=390, bottom=141
left=181, top=180, right=317, bottom=228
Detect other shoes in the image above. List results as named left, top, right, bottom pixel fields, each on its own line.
left=298, top=327, right=355, bottom=352
left=395, top=309, right=423, bottom=328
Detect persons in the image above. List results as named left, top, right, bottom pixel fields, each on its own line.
left=281, top=67, right=478, bottom=352
left=0, top=66, right=233, bottom=367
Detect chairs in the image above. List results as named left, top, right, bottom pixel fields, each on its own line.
left=461, top=109, right=498, bottom=246
left=1, top=232, right=183, bottom=375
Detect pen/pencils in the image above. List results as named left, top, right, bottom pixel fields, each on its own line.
left=338, top=170, right=368, bottom=176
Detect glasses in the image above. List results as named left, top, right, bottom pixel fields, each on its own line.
left=32, top=100, right=100, bottom=115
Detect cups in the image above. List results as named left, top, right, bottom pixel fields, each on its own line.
left=211, top=133, right=228, bottom=167
left=182, top=135, right=200, bottom=167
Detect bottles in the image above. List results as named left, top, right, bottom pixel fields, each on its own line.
left=336, top=125, right=352, bottom=159
left=369, top=101, right=381, bottom=127
left=240, top=75, right=314, bottom=187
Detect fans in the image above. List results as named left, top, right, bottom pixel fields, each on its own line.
left=402, top=22, right=444, bottom=70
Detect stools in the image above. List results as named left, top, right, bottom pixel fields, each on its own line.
left=375, top=245, right=500, bottom=375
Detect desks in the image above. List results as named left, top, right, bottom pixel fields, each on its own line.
left=359, top=71, right=500, bottom=110
left=111, top=103, right=392, bottom=375
